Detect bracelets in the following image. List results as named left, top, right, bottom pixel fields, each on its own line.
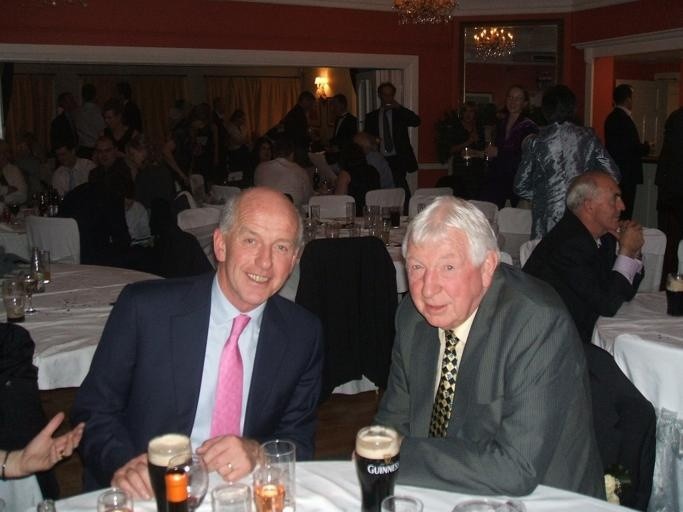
left=2, top=449, right=12, bottom=479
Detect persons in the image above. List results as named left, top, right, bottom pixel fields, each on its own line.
left=72, top=190, right=325, bottom=501
left=0, top=83, right=579, bottom=255
left=0, top=411, right=86, bottom=481
left=522, top=168, right=657, bottom=512
left=604, top=83, right=658, bottom=221
left=653, top=105, right=682, bottom=291
left=0, top=320, right=62, bottom=500
left=354, top=194, right=608, bottom=503
left=514, top=86, right=619, bottom=239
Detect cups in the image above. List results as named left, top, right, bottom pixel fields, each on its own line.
left=452, top=497, right=525, bottom=512
left=211, top=482, right=255, bottom=511
left=463, top=143, right=472, bottom=159
left=2, top=278, right=26, bottom=322
left=166, top=455, right=209, bottom=510
left=252, top=467, right=287, bottom=511
left=297, top=202, right=400, bottom=258
left=260, top=440, right=296, bottom=510
left=381, top=494, right=423, bottom=512
left=39, top=251, right=50, bottom=284
left=356, top=425, right=403, bottom=512
left=321, top=175, right=327, bottom=184
left=97, top=489, right=135, bottom=511
left=664, top=272, right=683, bottom=316
left=148, top=433, right=192, bottom=512
left=485, top=143, right=496, bottom=161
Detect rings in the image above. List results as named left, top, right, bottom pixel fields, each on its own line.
left=59, top=452, right=66, bottom=459
left=227, top=461, right=236, bottom=474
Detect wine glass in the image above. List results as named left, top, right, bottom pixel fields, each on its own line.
left=19, top=268, right=39, bottom=315
left=9, top=201, right=22, bottom=225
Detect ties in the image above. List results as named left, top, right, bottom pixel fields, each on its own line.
left=429, top=330, right=458, bottom=437
left=383, top=111, right=393, bottom=154
left=212, top=315, right=250, bottom=436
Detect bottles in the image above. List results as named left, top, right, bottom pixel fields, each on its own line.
left=30, top=247, right=45, bottom=293
left=37, top=499, right=56, bottom=512
left=312, top=168, right=320, bottom=191
left=38, top=184, right=59, bottom=217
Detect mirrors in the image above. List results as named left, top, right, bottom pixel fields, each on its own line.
left=457, top=20, right=566, bottom=109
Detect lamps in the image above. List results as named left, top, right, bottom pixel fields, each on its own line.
left=475, top=27, right=517, bottom=58
left=392, top=0, right=462, bottom=26
